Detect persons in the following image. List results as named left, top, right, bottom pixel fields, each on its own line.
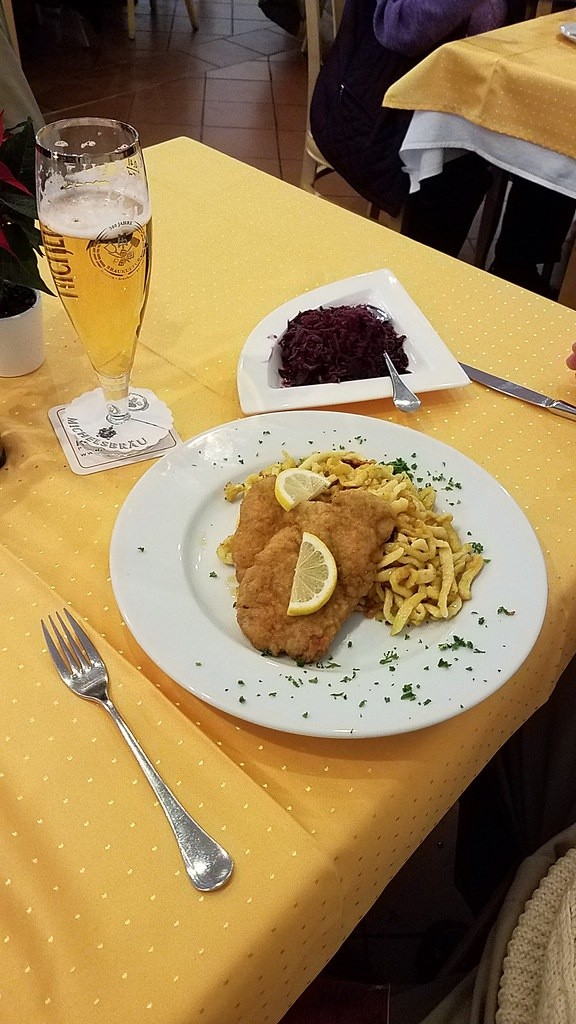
left=311, top=0, right=576, bottom=290
left=278, top=652, right=576, bottom=1023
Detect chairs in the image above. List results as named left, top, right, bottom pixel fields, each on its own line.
left=305, top=0, right=381, bottom=220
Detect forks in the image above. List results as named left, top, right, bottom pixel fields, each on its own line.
left=40, top=607, right=234, bottom=893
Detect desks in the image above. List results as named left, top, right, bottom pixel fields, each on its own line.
left=0, top=134, right=576, bottom=1024
left=381, top=7, right=576, bottom=312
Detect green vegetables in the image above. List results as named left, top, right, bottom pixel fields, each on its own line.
left=208, top=434, right=516, bottom=719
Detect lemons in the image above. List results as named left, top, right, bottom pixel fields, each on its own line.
left=274, top=468, right=332, bottom=512
left=287, top=532, right=337, bottom=616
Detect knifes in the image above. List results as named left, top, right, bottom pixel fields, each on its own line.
left=458, top=360, right=576, bottom=422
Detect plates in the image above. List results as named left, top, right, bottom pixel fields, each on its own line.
left=108, top=410, right=549, bottom=740
left=559, top=22, right=576, bottom=43
left=237, top=269, right=471, bottom=416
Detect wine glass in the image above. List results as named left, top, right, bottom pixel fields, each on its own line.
left=34, top=116, right=174, bottom=452
